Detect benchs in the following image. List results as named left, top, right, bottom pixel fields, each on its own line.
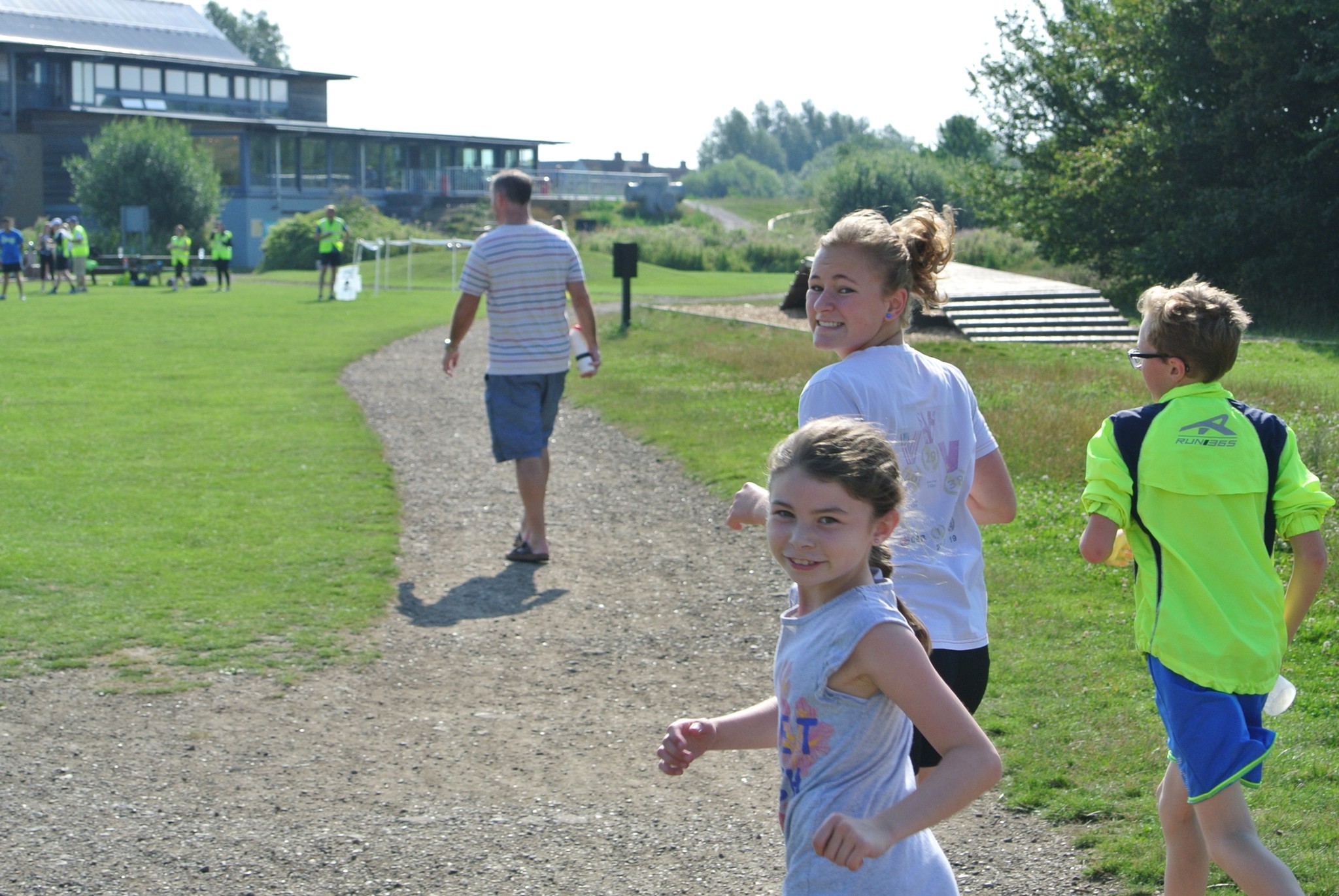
left=91, top=253, right=217, bottom=284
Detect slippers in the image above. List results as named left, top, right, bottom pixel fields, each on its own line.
left=513, top=533, right=524, bottom=547
left=506, top=541, right=549, bottom=561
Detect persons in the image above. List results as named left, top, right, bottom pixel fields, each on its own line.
left=315, top=206, right=350, bottom=305
left=656, top=415, right=1002, bottom=896
left=36, top=217, right=91, bottom=294
left=440, top=168, right=602, bottom=563
left=726, top=197, right=1017, bottom=788
left=166, top=224, right=194, bottom=294
left=1078, top=272, right=1337, bottom=896
left=0, top=216, right=28, bottom=301
left=551, top=212, right=568, bottom=234
left=207, top=224, right=234, bottom=292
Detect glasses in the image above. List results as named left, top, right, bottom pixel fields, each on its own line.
left=1127, top=347, right=1189, bottom=371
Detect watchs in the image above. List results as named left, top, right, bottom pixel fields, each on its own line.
left=443, top=337, right=459, bottom=353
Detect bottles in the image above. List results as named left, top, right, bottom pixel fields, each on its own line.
left=1263, top=674, right=1297, bottom=716
left=569, top=324, right=595, bottom=375
